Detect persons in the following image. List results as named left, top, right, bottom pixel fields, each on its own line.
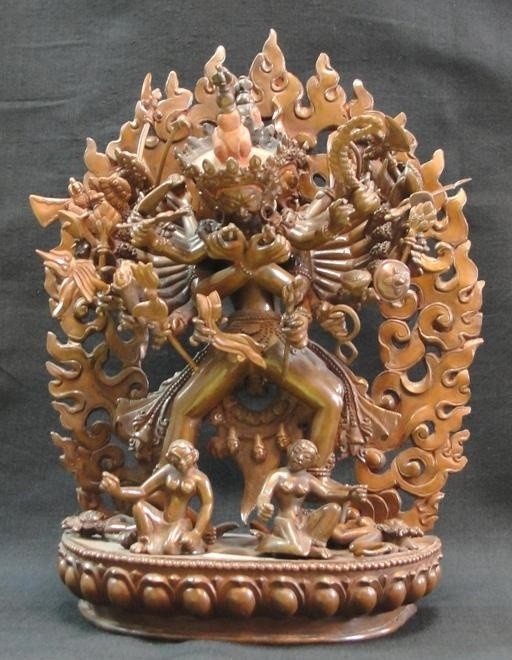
left=99, top=62, right=368, bottom=560
